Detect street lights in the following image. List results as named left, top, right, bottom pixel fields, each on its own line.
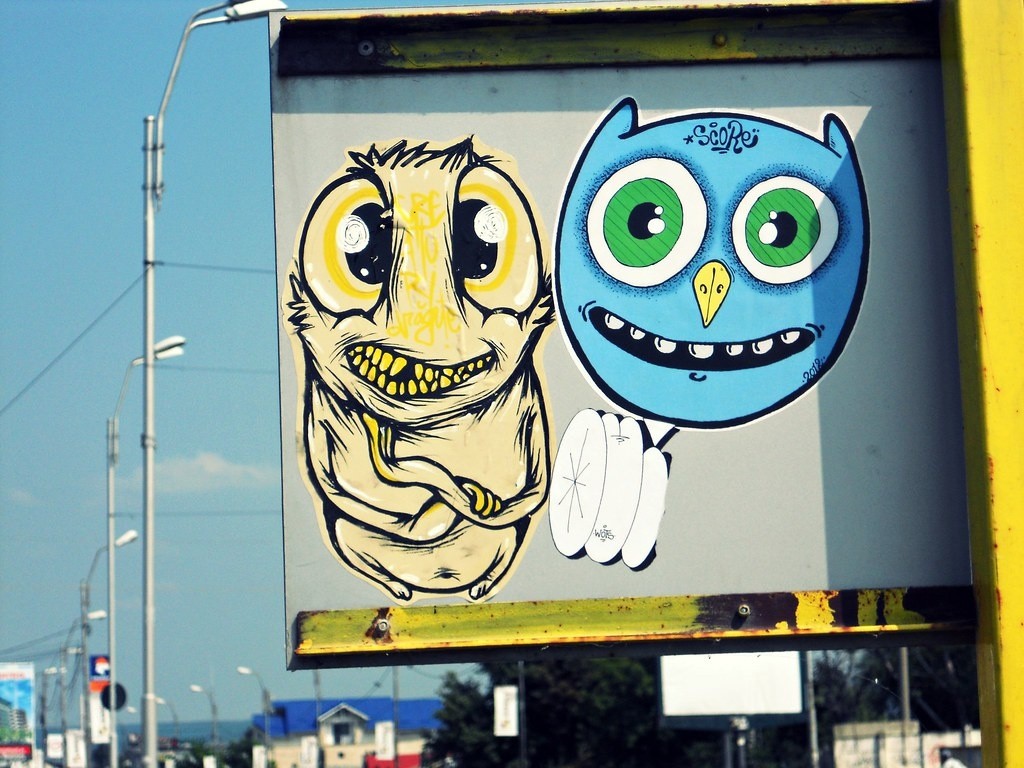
left=84, top=527, right=139, bottom=768
left=107, top=335, right=189, bottom=768
left=190, top=683, right=217, bottom=758
left=41, top=666, right=69, bottom=763
left=59, top=609, right=108, bottom=768
left=139, top=0, right=290, bottom=768
left=237, top=666, right=272, bottom=768
left=155, top=698, right=181, bottom=754
